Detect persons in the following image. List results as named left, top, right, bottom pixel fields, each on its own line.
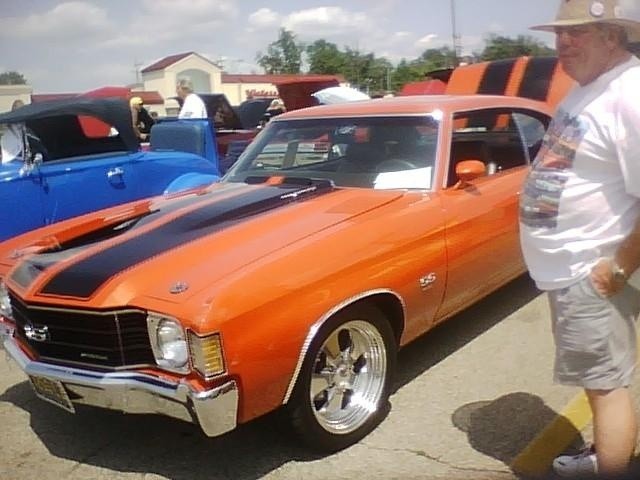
left=517, top=0, right=640, bottom=479
left=109, top=96, right=148, bottom=141
left=214, top=103, right=233, bottom=156
left=264, top=99, right=285, bottom=122
left=176, top=79, right=209, bottom=119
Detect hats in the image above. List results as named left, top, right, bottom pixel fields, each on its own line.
left=528, top=0, right=640, bottom=44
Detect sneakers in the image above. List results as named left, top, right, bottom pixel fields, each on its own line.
left=553, top=443, right=635, bottom=479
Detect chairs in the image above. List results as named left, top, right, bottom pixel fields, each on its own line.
left=337, top=136, right=533, bottom=188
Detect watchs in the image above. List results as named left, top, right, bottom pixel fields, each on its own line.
left=611, top=258, right=629, bottom=283
